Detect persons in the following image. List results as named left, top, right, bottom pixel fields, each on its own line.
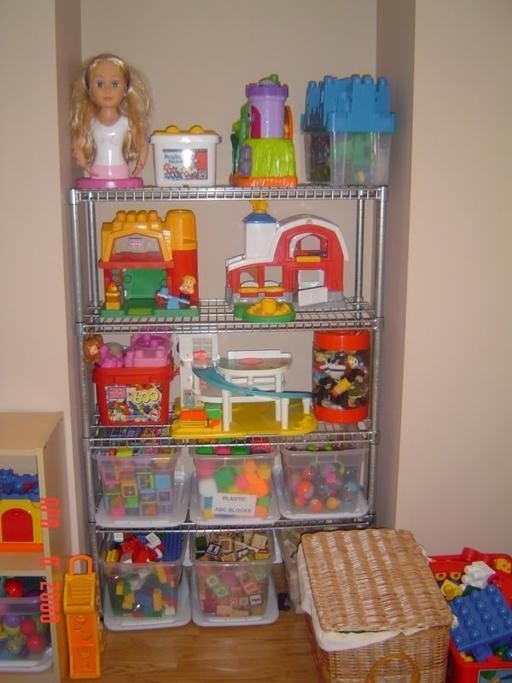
left=72, top=54, right=149, bottom=179
left=85, top=336, right=122, bottom=368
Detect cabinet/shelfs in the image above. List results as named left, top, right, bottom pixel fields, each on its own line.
left=0, top=411, right=72, bottom=683
left=67, top=185, right=388, bottom=572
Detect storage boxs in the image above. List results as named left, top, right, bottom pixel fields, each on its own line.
left=190, top=533, right=276, bottom=621
left=99, top=534, right=188, bottom=624
left=428, top=553, right=512, bottom=683
left=92, top=366, right=175, bottom=424
left=304, top=132, right=393, bottom=188
left=92, top=442, right=370, bottom=522
left=0, top=596, right=52, bottom=672
